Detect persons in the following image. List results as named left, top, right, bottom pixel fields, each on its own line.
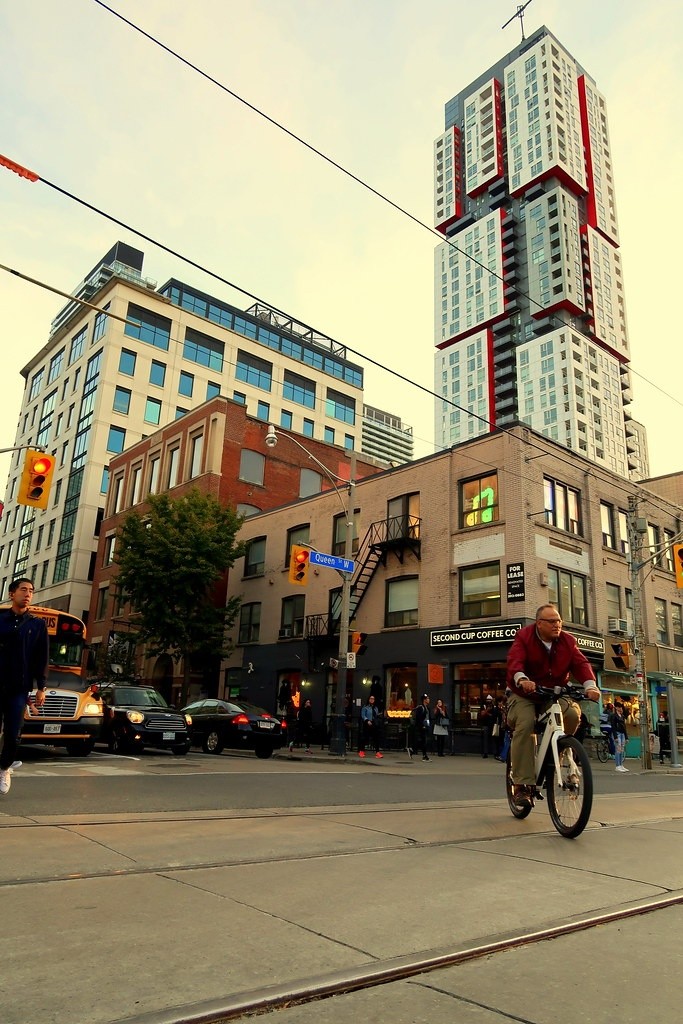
left=573, top=709, right=592, bottom=765
left=357, top=695, right=384, bottom=758
left=649, top=712, right=671, bottom=764
left=599, top=703, right=617, bottom=759
left=289, top=699, right=314, bottom=755
left=431, top=698, right=451, bottom=758
left=0, top=577, right=51, bottom=794
left=610, top=702, right=630, bottom=773
left=476, top=693, right=512, bottom=763
left=406, top=694, right=433, bottom=762
left=504, top=604, right=601, bottom=808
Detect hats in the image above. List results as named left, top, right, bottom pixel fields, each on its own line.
left=421, top=693, right=428, bottom=701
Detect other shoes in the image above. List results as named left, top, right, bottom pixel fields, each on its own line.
left=407, top=748, right=413, bottom=759
left=496, top=756, right=505, bottom=763
left=304, top=748, right=313, bottom=755
left=422, top=756, right=432, bottom=761
left=483, top=754, right=488, bottom=758
left=358, top=751, right=366, bottom=757
left=438, top=753, right=444, bottom=757
left=10, top=761, right=22, bottom=769
left=376, top=752, right=383, bottom=758
left=289, top=745, right=292, bottom=751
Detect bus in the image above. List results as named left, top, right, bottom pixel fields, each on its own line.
left=0, top=604, right=124, bottom=757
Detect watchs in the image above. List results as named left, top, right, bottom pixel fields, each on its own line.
left=37, top=687, right=47, bottom=691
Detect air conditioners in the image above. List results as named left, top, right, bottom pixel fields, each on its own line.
left=279, top=629, right=292, bottom=637
left=609, top=618, right=628, bottom=633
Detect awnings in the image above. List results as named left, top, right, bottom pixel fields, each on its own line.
left=645, top=670, right=683, bottom=690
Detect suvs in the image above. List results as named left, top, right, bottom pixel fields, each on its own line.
left=90, top=683, right=192, bottom=757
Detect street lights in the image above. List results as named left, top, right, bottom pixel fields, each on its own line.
left=265, top=423, right=354, bottom=757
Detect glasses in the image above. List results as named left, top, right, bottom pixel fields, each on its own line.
left=538, top=619, right=562, bottom=624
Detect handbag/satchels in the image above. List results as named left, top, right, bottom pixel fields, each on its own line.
left=492, top=717, right=500, bottom=737
left=440, top=713, right=449, bottom=725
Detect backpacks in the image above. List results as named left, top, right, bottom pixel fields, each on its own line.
left=411, top=705, right=428, bottom=723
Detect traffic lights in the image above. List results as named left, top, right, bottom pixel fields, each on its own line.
left=610, top=642, right=629, bottom=670
left=288, top=544, right=309, bottom=586
left=17, top=449, right=56, bottom=510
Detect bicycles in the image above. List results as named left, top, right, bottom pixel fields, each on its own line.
left=506, top=684, right=600, bottom=840
left=596, top=730, right=626, bottom=763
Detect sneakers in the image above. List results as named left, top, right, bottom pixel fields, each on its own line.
left=0, top=769, right=11, bottom=794
left=513, top=786, right=532, bottom=806
left=622, top=765, right=629, bottom=772
left=616, top=765, right=625, bottom=773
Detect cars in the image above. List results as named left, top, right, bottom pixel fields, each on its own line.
left=180, top=697, right=286, bottom=759
